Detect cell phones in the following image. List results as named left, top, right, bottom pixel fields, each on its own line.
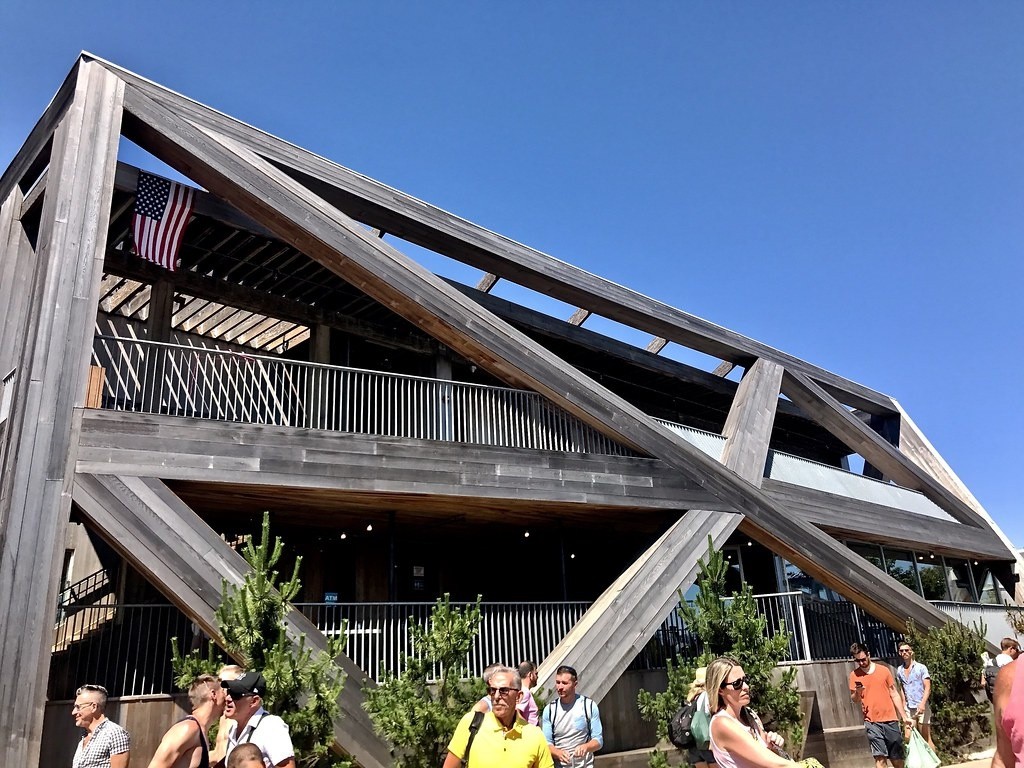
left=855, top=681, right=863, bottom=687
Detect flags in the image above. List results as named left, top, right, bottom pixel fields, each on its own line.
left=130, top=171, right=197, bottom=272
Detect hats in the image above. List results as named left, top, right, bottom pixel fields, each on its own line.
left=221, top=671, right=266, bottom=697
left=693, top=667, right=709, bottom=685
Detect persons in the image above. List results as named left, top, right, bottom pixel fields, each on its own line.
left=706, top=657, right=804, bottom=768
left=687, top=667, right=719, bottom=768
left=72, top=684, right=131, bottom=768
left=849, top=643, right=916, bottom=768
left=228, top=743, right=267, bottom=768
left=148, top=674, right=226, bottom=768
left=542, top=665, right=603, bottom=768
left=472, top=662, right=505, bottom=713
left=897, top=642, right=936, bottom=753
left=516, top=661, right=540, bottom=728
left=992, top=651, right=1024, bottom=768
left=209, top=665, right=244, bottom=768
left=981, top=633, right=1019, bottom=701
left=444, top=667, right=554, bottom=768
left=221, top=672, right=296, bottom=768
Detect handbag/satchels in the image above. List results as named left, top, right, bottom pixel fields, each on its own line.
left=745, top=706, right=792, bottom=761
left=689, top=692, right=709, bottom=751
left=902, top=728, right=942, bottom=768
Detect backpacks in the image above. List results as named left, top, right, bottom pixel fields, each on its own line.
left=984, top=658, right=1000, bottom=705
left=667, top=694, right=697, bottom=750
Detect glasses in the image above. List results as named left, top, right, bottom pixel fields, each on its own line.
left=723, top=676, right=751, bottom=690
left=899, top=649, right=911, bottom=652
left=854, top=655, right=867, bottom=663
left=486, top=687, right=520, bottom=697
left=557, top=665, right=578, bottom=682
left=226, top=688, right=257, bottom=700
left=72, top=702, right=100, bottom=711
left=1011, top=647, right=1020, bottom=654
